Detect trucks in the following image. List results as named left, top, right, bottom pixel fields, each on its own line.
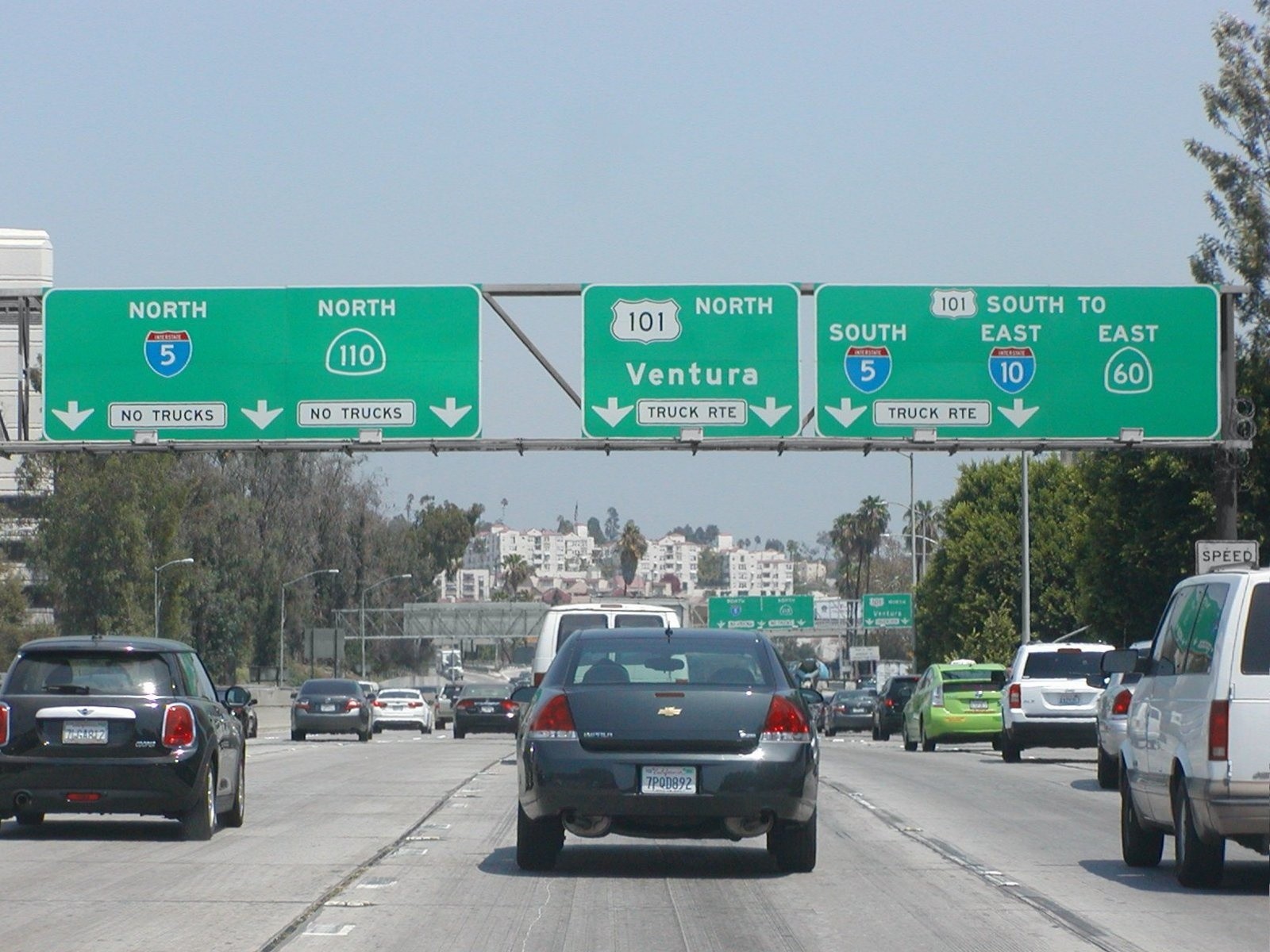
left=435, top=649, right=463, bottom=681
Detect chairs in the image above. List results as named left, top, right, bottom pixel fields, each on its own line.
left=698, top=664, right=758, bottom=685
left=578, top=656, right=636, bottom=688
left=38, top=657, right=75, bottom=690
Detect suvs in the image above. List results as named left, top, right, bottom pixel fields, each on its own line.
left=1100, top=559, right=1270, bottom=889
left=991, top=640, right=1116, bottom=762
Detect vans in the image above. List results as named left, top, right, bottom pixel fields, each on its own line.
left=532, top=603, right=689, bottom=685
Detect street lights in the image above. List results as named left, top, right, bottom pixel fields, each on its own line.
left=361, top=574, right=411, bottom=679
left=280, top=569, right=339, bottom=689
left=874, top=501, right=926, bottom=579
left=155, top=559, right=194, bottom=640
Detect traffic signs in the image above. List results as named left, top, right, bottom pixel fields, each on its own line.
left=862, top=594, right=913, bottom=629
left=708, top=595, right=814, bottom=630
left=39, top=286, right=482, bottom=443
left=815, top=284, right=1220, bottom=440
left=582, top=284, right=799, bottom=439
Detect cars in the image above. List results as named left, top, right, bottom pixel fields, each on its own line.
left=898, top=660, right=1011, bottom=751
left=374, top=688, right=433, bottom=734
left=358, top=671, right=531, bottom=729
left=0, top=634, right=252, bottom=842
left=450, top=682, right=518, bottom=739
left=290, top=679, right=376, bottom=741
left=808, top=675, right=925, bottom=740
left=215, top=690, right=258, bottom=739
left=1086, top=640, right=1155, bottom=788
left=510, top=628, right=823, bottom=873
